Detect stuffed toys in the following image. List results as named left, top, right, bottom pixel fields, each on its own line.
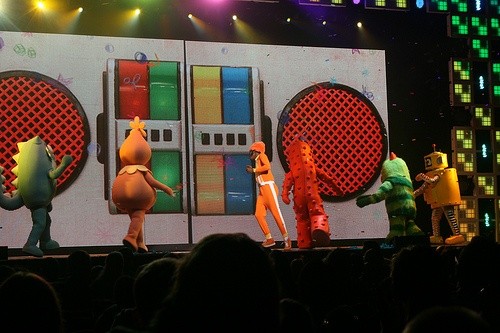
left=281, top=139, right=345, bottom=248
left=355, top=151, right=426, bottom=241
left=0, top=136, right=72, bottom=259
left=111, top=114, right=174, bottom=251
left=412, top=144, right=466, bottom=246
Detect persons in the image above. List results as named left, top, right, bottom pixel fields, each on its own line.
left=245, top=141, right=293, bottom=251
left=0, top=234, right=500, bottom=333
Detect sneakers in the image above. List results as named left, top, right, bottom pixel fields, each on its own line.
left=260, top=238, right=275, bottom=246
left=283, top=235, right=291, bottom=250
